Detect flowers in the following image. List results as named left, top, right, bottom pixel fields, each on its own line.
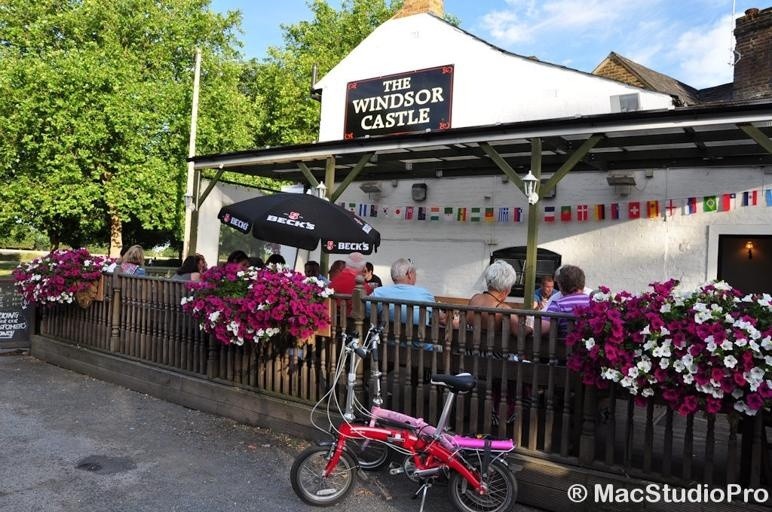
left=12, top=247, right=118, bottom=310
left=563, top=278, right=772, bottom=417
left=180, top=262, right=335, bottom=346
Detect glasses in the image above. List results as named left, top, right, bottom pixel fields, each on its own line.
left=406, top=256, right=416, bottom=274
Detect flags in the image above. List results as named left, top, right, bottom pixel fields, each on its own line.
left=359, top=204, right=367, bottom=217
left=545, top=207, right=555, bottom=221
left=514, top=207, right=524, bottom=223
left=370, top=205, right=378, bottom=217
left=382, top=206, right=390, bottom=217
left=765, top=190, right=772, bottom=206
left=485, top=208, right=495, bottom=223
left=393, top=206, right=403, bottom=219
left=561, top=206, right=571, bottom=222
left=337, top=202, right=346, bottom=208
left=498, top=208, right=509, bottom=224
left=578, top=205, right=588, bottom=221
left=471, top=208, right=481, bottom=223
left=722, top=193, right=736, bottom=211
left=431, top=208, right=440, bottom=220
left=743, top=191, right=757, bottom=206
left=665, top=199, right=677, bottom=216
left=628, top=201, right=640, bottom=218
left=703, top=196, right=717, bottom=211
left=348, top=203, right=355, bottom=213
left=405, top=207, right=414, bottom=220
left=595, top=204, right=604, bottom=221
left=684, top=198, right=696, bottom=214
left=611, top=202, right=624, bottom=220
left=647, top=200, right=658, bottom=218
left=457, top=208, right=466, bottom=222
left=418, top=207, right=427, bottom=220
left=443, top=208, right=453, bottom=221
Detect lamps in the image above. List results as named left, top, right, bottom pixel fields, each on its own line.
left=412, top=183, right=428, bottom=202
left=315, top=181, right=329, bottom=201
left=745, top=241, right=754, bottom=259
left=359, top=182, right=383, bottom=202
left=521, top=170, right=539, bottom=205
left=607, top=176, right=637, bottom=196
left=540, top=178, right=557, bottom=200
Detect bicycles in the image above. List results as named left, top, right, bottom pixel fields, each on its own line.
left=289, top=320, right=519, bottom=512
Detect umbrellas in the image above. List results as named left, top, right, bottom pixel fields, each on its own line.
left=219, top=185, right=380, bottom=270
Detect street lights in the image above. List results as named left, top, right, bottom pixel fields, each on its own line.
left=183, top=169, right=203, bottom=253
left=315, top=159, right=335, bottom=274
left=521, top=139, right=541, bottom=311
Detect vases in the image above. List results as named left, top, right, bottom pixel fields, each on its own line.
left=74, top=274, right=103, bottom=308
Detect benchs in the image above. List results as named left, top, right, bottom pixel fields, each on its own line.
left=325, top=314, right=577, bottom=391
left=433, top=296, right=525, bottom=310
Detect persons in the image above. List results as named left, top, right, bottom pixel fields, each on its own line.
left=466, top=260, right=531, bottom=337
left=366, top=258, right=463, bottom=328
left=362, top=263, right=382, bottom=287
left=534, top=276, right=558, bottom=310
left=328, top=261, right=344, bottom=281
left=330, top=252, right=374, bottom=315
left=540, top=267, right=592, bottom=336
left=303, top=262, right=330, bottom=286
left=543, top=265, right=602, bottom=311
left=171, top=254, right=207, bottom=280
left=119, top=245, right=146, bottom=276
left=265, top=254, right=286, bottom=269
left=226, top=251, right=248, bottom=270
